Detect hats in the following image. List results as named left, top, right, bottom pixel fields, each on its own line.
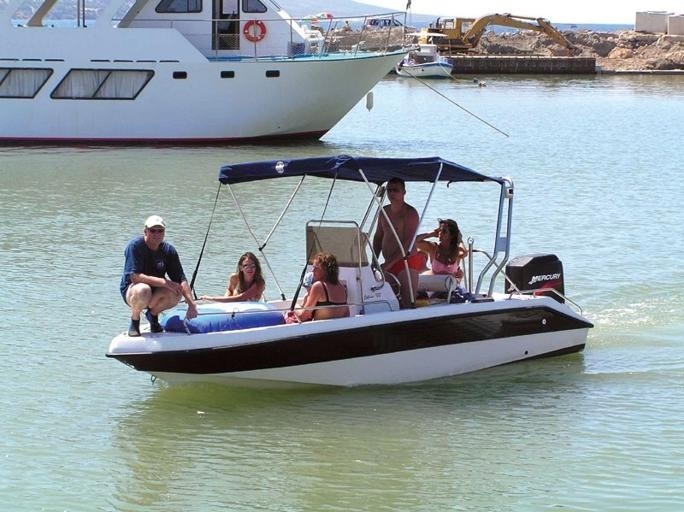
left=144, top=215, right=167, bottom=228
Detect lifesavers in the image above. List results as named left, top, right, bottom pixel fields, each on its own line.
left=243, top=19, right=266, bottom=41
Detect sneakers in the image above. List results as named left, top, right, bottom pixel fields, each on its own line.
left=127, top=315, right=142, bottom=338
left=144, top=308, right=163, bottom=333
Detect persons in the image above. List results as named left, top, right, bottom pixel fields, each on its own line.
left=199, top=251, right=266, bottom=303
left=283, top=252, right=350, bottom=324
left=345, top=21, right=349, bottom=28
left=415, top=218, right=468, bottom=300
left=120, top=215, right=198, bottom=337
left=371, top=178, right=419, bottom=309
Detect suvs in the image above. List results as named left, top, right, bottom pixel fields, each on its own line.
left=364, top=17, right=416, bottom=30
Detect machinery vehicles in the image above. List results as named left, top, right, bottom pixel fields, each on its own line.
left=420, top=15, right=580, bottom=57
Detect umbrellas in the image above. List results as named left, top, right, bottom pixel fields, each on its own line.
left=316, top=12, right=334, bottom=20
left=297, top=16, right=320, bottom=23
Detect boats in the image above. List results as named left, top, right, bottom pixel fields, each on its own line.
left=0, top=0, right=414, bottom=145
left=394, top=32, right=455, bottom=80
left=105, top=155, right=594, bottom=391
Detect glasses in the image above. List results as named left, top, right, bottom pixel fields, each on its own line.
left=240, top=262, right=256, bottom=270
left=150, top=229, right=165, bottom=233
